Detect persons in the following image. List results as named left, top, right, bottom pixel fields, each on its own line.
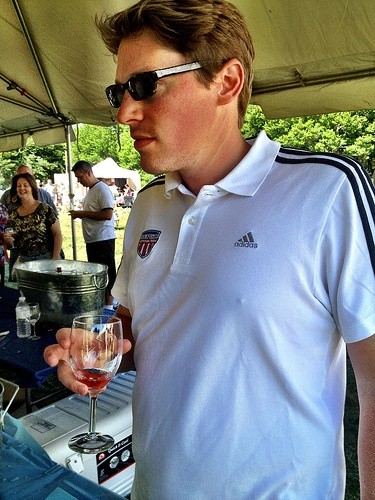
left=4, top=173, right=62, bottom=281
left=1, top=201, right=9, bottom=300
left=68, top=160, right=117, bottom=292
left=2, top=165, right=66, bottom=282
left=41, top=177, right=138, bottom=205
left=44, top=0, right=375, bottom=499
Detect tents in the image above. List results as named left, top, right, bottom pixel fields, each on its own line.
left=77, top=158, right=141, bottom=208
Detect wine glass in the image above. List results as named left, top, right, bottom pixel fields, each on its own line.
left=67, top=315, right=123, bottom=454
left=5, top=228, right=17, bottom=249
left=25, top=303, right=41, bottom=340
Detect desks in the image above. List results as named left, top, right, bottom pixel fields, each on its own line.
left=0, top=283, right=74, bottom=414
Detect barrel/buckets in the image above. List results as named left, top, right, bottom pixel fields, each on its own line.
left=13, top=260, right=109, bottom=325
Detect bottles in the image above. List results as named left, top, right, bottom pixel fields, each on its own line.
left=55, top=268, right=62, bottom=275
left=15, top=297, right=31, bottom=338
left=70, top=270, right=77, bottom=275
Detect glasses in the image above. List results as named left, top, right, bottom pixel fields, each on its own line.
left=106, top=60, right=207, bottom=106
